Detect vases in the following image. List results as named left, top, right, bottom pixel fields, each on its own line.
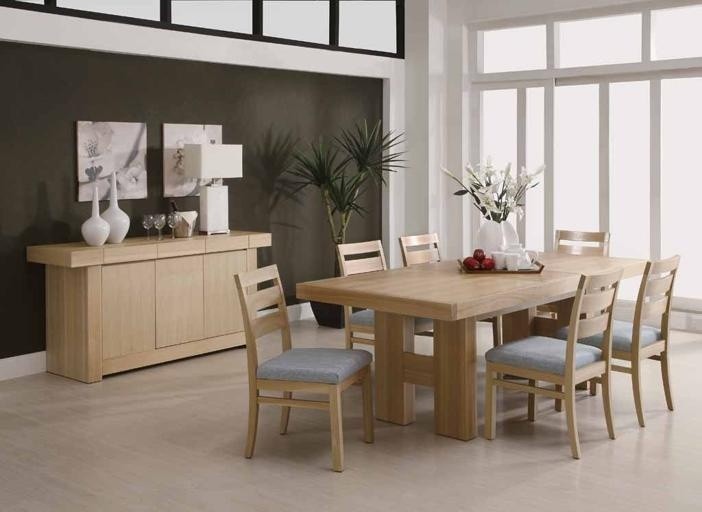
left=473, top=221, right=518, bottom=256
left=82, top=187, right=108, bottom=245
left=101, top=171, right=130, bottom=241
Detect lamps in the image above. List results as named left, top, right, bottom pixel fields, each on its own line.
left=182, top=145, right=243, bottom=235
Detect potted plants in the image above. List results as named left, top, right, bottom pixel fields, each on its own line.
left=256, top=120, right=408, bottom=329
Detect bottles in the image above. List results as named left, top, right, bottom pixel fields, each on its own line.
left=171, top=201, right=180, bottom=212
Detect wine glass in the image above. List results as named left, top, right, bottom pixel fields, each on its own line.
left=142, top=214, right=154, bottom=241
left=168, top=215, right=179, bottom=240
left=153, top=214, right=166, bottom=240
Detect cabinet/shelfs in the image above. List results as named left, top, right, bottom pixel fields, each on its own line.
left=25, top=226, right=272, bottom=383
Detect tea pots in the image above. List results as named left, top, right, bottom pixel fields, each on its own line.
left=498, top=243, right=537, bottom=268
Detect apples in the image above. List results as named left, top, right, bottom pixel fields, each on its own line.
left=463, top=249, right=494, bottom=270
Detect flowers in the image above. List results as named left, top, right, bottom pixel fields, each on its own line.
left=438, top=157, right=543, bottom=221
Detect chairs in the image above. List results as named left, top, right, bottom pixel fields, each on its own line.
left=234, top=263, right=374, bottom=474
left=532, top=228, right=610, bottom=336
left=560, top=254, right=680, bottom=426
left=335, top=238, right=402, bottom=351
left=485, top=266, right=624, bottom=459
left=400, top=232, right=447, bottom=335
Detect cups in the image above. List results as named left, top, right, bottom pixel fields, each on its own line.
left=505, top=253, right=519, bottom=272
left=492, top=252, right=505, bottom=270
